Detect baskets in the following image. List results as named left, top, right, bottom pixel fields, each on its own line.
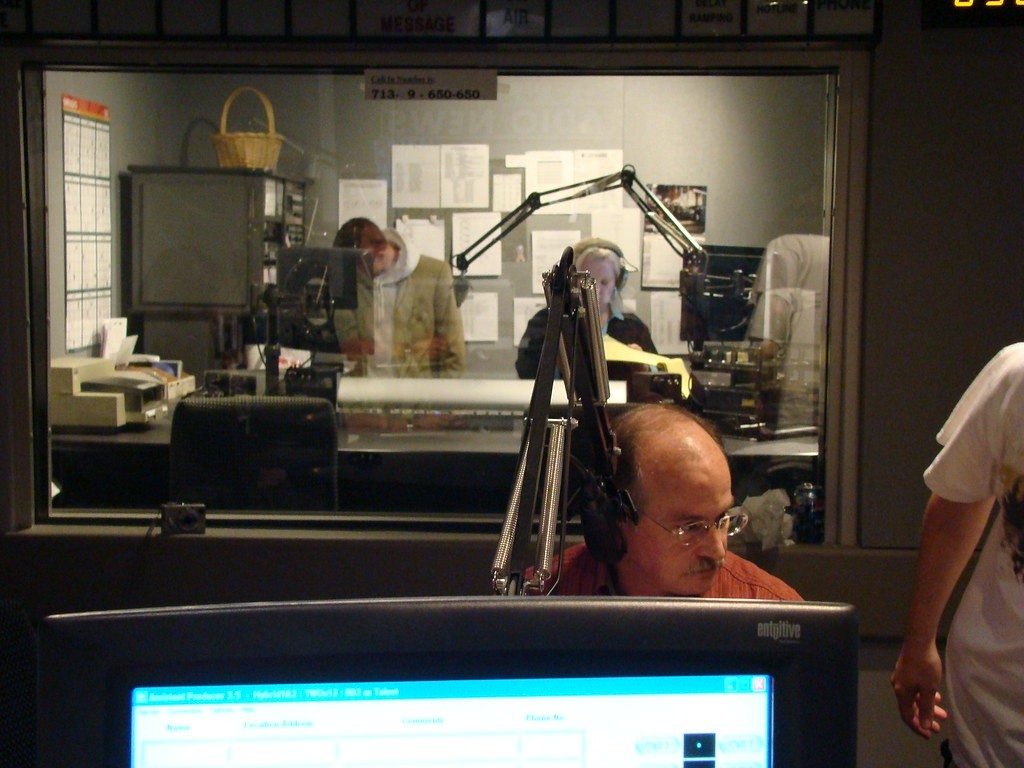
left=210, top=87, right=286, bottom=170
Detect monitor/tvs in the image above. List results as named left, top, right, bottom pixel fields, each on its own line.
left=679, top=245, right=767, bottom=341
left=46, top=599, right=862, bottom=768
left=275, top=247, right=358, bottom=309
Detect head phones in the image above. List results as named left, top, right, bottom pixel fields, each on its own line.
left=574, top=239, right=628, bottom=291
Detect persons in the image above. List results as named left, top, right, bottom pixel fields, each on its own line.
left=891, top=341, right=1024, bottom=768
left=515, top=238, right=659, bottom=380
left=524, top=404, right=805, bottom=601
left=745, top=231, right=829, bottom=438
left=332, top=217, right=465, bottom=379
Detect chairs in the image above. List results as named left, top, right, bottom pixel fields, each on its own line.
left=167, top=394, right=338, bottom=511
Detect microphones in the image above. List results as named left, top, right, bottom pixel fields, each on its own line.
left=454, top=275, right=469, bottom=308
left=568, top=458, right=627, bottom=564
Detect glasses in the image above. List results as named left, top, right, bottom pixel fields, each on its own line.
left=620, top=502, right=749, bottom=545
